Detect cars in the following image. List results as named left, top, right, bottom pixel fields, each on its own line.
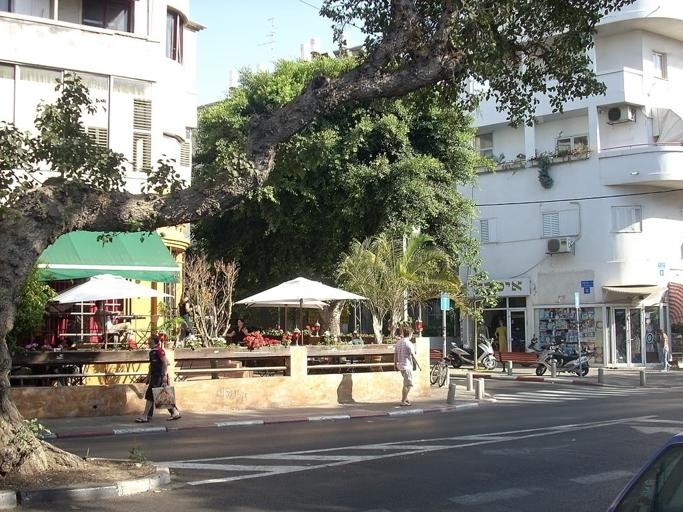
left=607, top=431, right=683, bottom=512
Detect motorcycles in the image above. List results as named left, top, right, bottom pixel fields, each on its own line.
left=446, top=332, right=497, bottom=370
left=522, top=333, right=596, bottom=376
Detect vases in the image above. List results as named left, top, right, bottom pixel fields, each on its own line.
left=31, top=350, right=49, bottom=361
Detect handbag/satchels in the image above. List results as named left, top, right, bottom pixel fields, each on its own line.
left=151, top=383, right=175, bottom=409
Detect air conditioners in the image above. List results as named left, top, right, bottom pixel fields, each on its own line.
left=605, top=106, right=636, bottom=125
left=545, top=237, right=576, bottom=256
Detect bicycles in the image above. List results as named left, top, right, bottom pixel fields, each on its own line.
left=430, top=349, right=456, bottom=388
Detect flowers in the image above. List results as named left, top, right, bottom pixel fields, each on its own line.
left=26, top=343, right=51, bottom=352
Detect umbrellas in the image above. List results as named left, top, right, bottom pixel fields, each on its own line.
left=236, top=275, right=370, bottom=345
left=46, top=274, right=177, bottom=334
left=246, top=300, right=330, bottom=328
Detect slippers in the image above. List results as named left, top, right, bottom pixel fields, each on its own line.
left=134, top=411, right=182, bottom=423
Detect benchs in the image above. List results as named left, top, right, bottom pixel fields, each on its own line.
left=430, top=348, right=443, bottom=361
left=493, top=352, right=544, bottom=372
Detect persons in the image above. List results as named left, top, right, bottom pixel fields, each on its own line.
left=134, top=332, right=181, bottom=422
left=477, top=319, right=490, bottom=340
left=393, top=326, right=422, bottom=407
left=178, top=290, right=198, bottom=343
left=92, top=300, right=134, bottom=350
left=225, top=318, right=251, bottom=346
left=144, top=345, right=180, bottom=421
left=494, top=318, right=508, bottom=353
left=655, top=327, right=672, bottom=374
